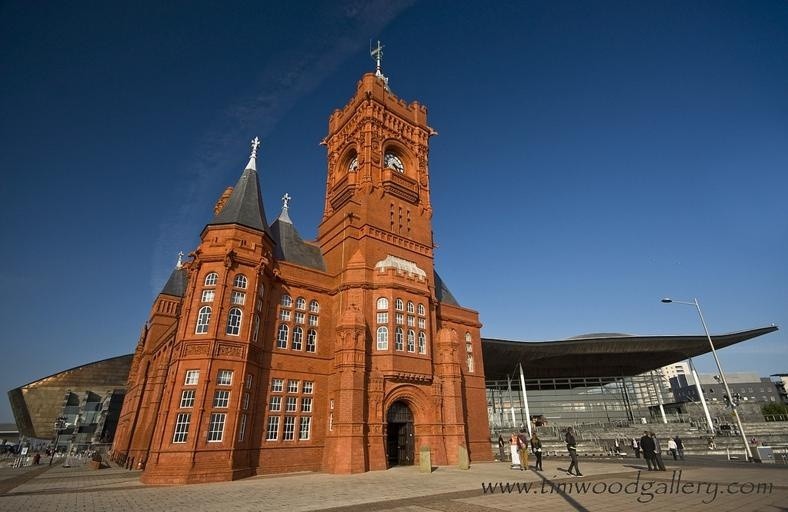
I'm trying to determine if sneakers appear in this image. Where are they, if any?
[648,467,666,471]
[567,471,583,477]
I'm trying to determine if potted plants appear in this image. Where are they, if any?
[90,455,102,468]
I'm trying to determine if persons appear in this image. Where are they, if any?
[529,433,543,471]
[498,434,507,462]
[565,427,584,477]
[610,416,740,473]
[516,429,528,471]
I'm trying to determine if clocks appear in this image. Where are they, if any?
[384,152,404,174]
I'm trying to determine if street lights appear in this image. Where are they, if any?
[660,297,756,464]
